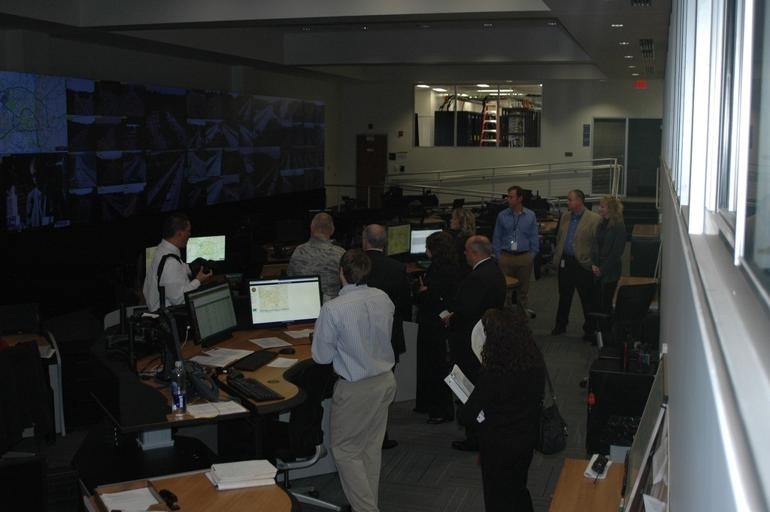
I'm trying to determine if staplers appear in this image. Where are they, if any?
[158,489,180,510]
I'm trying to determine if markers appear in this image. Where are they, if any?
[619,450,630,512]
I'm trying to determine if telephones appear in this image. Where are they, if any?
[186,370,219,402]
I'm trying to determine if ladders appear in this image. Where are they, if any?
[479,95,500,147]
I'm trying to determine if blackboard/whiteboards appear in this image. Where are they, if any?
[619,353,670,512]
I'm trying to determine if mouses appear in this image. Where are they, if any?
[279,347,295,354]
[227,370,242,380]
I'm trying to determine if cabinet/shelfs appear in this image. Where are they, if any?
[433,105,540,147]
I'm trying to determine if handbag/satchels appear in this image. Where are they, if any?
[535,404,566,453]
[157,304,191,340]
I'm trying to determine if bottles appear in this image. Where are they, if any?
[621,341,630,371]
[169,361,188,416]
[636,344,652,375]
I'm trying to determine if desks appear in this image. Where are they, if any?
[544,455,627,512]
[78,461,306,512]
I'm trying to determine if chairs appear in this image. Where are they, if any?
[267,364,344,512]
[583,281,658,347]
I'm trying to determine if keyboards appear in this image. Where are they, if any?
[228,379,285,401]
[235,350,277,370]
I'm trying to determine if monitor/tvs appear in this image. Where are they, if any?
[184,233,227,264]
[247,274,322,328]
[184,280,239,348]
[162,306,185,373]
[384,223,410,256]
[410,226,443,254]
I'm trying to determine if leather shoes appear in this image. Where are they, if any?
[552,326,566,335]
[428,417,450,424]
[452,440,479,450]
[382,439,399,449]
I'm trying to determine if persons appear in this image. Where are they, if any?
[582,194,627,346]
[548,189,607,337]
[492,186,540,323]
[24,155,63,228]
[285,204,552,512]
[141,212,214,320]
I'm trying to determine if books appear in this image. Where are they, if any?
[182,398,253,419]
